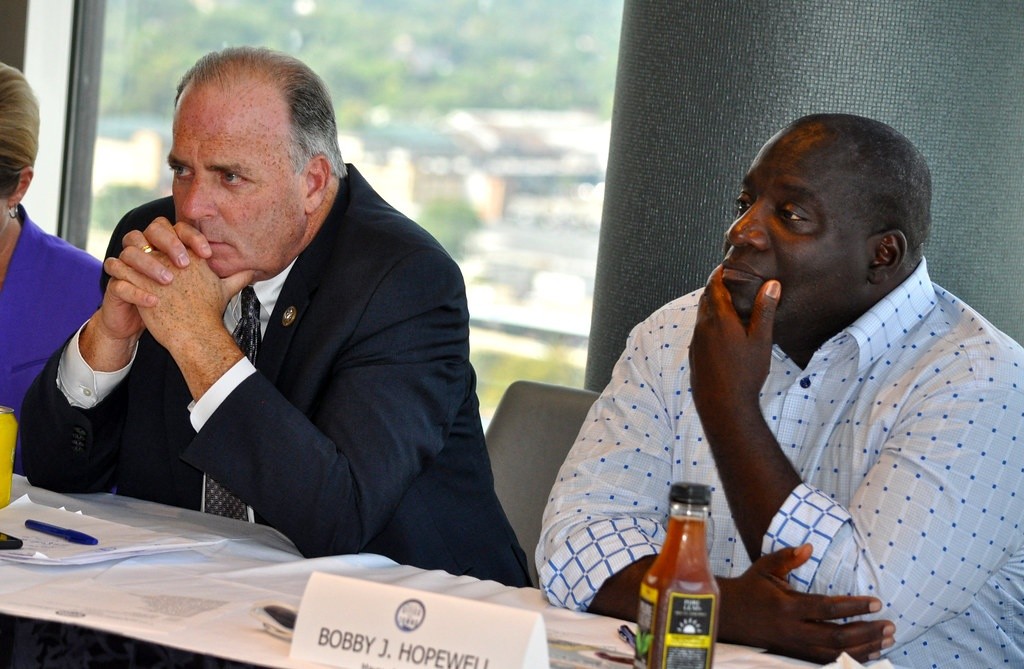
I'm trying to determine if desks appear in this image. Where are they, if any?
[0,465,904,669]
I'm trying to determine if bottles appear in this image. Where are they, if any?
[632,482,721,669]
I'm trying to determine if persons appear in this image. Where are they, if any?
[15,43,535,589]
[0,58,106,478]
[534,112,1024,669]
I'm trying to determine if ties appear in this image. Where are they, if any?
[205,286,261,522]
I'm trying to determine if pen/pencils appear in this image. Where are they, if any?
[25,519,98,546]
[617,623,635,647]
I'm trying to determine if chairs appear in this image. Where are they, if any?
[484,380,602,591]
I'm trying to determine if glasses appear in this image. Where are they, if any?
[250,599,298,640]
[0,165,27,199]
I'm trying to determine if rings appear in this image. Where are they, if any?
[141,246,153,253]
